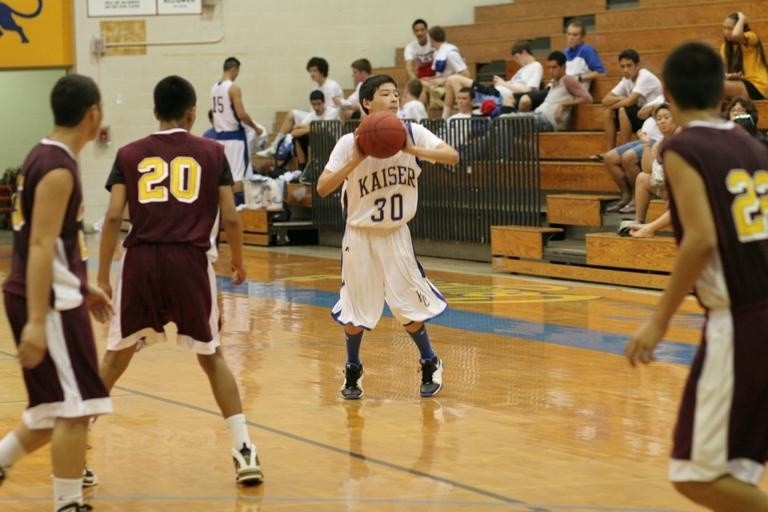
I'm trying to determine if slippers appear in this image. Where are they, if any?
[619,202,636,214]
[606,203,625,214]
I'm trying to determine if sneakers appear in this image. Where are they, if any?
[56,501,92,512]
[81,462,97,487]
[230,442,265,486]
[339,362,367,400]
[416,354,444,398]
[256,143,281,158]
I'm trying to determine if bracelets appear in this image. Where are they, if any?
[578,73,582,82]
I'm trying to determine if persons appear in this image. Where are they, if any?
[503,20,608,112]
[590,49,663,160]
[419,26,470,109]
[604,94,665,214]
[333,58,371,120]
[404,19,437,88]
[317,74,459,399]
[202,109,217,139]
[720,11,768,100]
[725,96,768,146]
[82,75,263,486]
[461,51,593,162]
[290,90,342,171]
[626,42,768,511]
[443,42,543,120]
[256,57,343,157]
[1,75,115,511]
[397,80,429,124]
[619,104,682,237]
[629,208,672,237]
[209,58,263,205]
[446,88,472,140]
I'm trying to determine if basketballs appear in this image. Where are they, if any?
[357,110,405,159]
